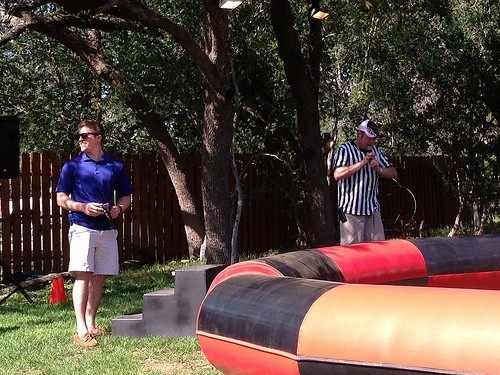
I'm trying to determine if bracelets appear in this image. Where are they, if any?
[379,167,383,175]
[119,205,122,214]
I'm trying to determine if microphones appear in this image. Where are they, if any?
[103,203,112,212]
[367,146,372,153]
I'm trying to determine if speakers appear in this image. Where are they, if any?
[0,115,20,179]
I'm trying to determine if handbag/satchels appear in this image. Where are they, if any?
[50,277,67,304]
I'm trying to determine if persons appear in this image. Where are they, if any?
[56,120,132,348]
[320,119,399,245]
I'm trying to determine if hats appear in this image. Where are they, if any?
[322,133,334,141]
[355,120,379,138]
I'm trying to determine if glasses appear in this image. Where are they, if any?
[76,133,100,141]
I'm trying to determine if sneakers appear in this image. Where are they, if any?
[73,332,98,347]
[90,328,106,337]
